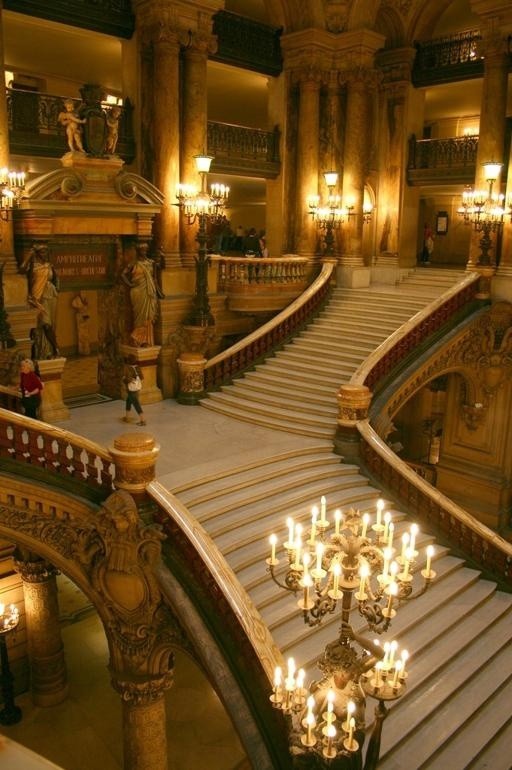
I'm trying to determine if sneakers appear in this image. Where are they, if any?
[136,421,146,426]
[120,417,130,423]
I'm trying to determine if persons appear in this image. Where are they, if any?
[120,355,147,427]
[0,235,18,346]
[421,222,437,264]
[19,242,63,359]
[13,359,43,418]
[104,107,121,154]
[119,241,166,347]
[59,99,86,153]
[235,225,272,285]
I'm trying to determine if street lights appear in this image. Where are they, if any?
[0,603,28,725]
[1,164,26,348]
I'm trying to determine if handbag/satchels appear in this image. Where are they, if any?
[22,396,41,408]
[128,376,142,392]
